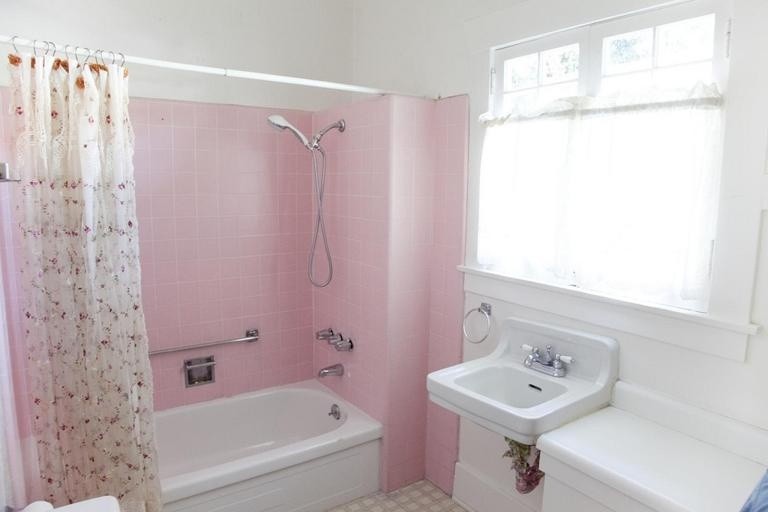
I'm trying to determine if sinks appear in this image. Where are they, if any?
[425,316,619,444]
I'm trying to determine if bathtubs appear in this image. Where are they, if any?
[154,389,348,512]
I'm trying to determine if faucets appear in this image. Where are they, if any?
[519,344,573,377]
[318,364,344,378]
